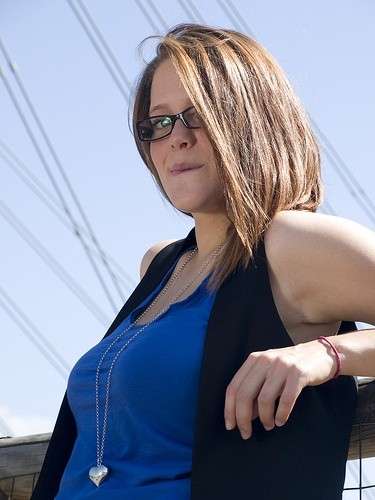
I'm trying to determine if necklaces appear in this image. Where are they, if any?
[88,233,230,488]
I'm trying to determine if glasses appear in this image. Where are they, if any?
[135,105,205,142]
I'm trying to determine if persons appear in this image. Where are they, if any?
[30,24,375,500]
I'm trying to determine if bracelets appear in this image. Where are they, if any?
[317,334,340,379]
